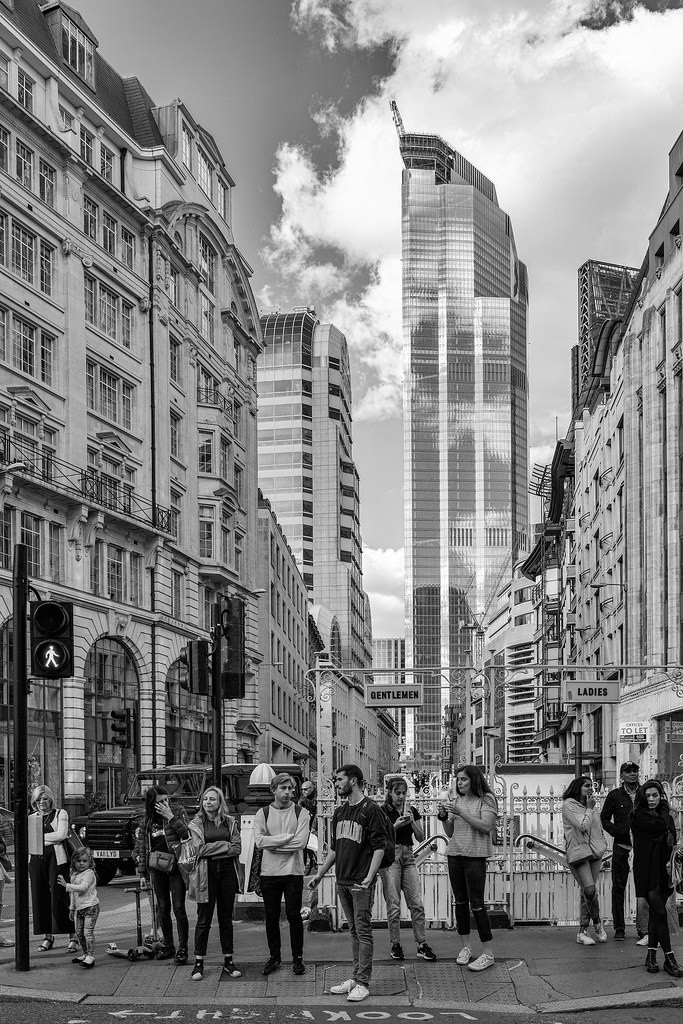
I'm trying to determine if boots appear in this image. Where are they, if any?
[645,948,683,977]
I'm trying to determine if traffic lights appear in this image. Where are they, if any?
[177,639,208,696]
[30,599,75,681]
[111,708,131,748]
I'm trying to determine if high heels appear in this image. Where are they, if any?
[37,934,55,952]
[66,938,80,953]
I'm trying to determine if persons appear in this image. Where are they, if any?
[379,778,436,962]
[252,772,312,975]
[56,846,101,968]
[626,782,683,977]
[29,784,80,954]
[636,779,680,946]
[561,776,607,946]
[298,780,317,869]
[137,785,189,966]
[0,807,16,947]
[306,764,387,1002]
[436,765,499,972]
[600,761,647,942]
[186,786,242,980]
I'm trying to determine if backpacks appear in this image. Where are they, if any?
[366,800,396,870]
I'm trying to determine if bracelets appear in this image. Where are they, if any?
[437,811,449,821]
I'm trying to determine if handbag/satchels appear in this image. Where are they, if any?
[0,844,13,872]
[149,851,175,873]
[177,837,197,891]
[567,843,594,866]
[63,829,84,864]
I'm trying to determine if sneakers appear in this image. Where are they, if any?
[416,943,436,960]
[456,947,495,971]
[390,942,405,959]
[330,979,369,1002]
[262,956,306,974]
[576,917,607,945]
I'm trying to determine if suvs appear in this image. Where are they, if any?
[74,763,304,887]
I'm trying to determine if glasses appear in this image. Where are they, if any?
[301,787,311,790]
[626,770,636,773]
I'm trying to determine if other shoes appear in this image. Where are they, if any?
[223,956,242,978]
[636,934,649,946]
[0,934,15,948]
[613,930,626,941]
[153,944,189,964]
[191,961,204,981]
[72,954,95,968]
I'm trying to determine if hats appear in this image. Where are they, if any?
[621,760,639,772]
